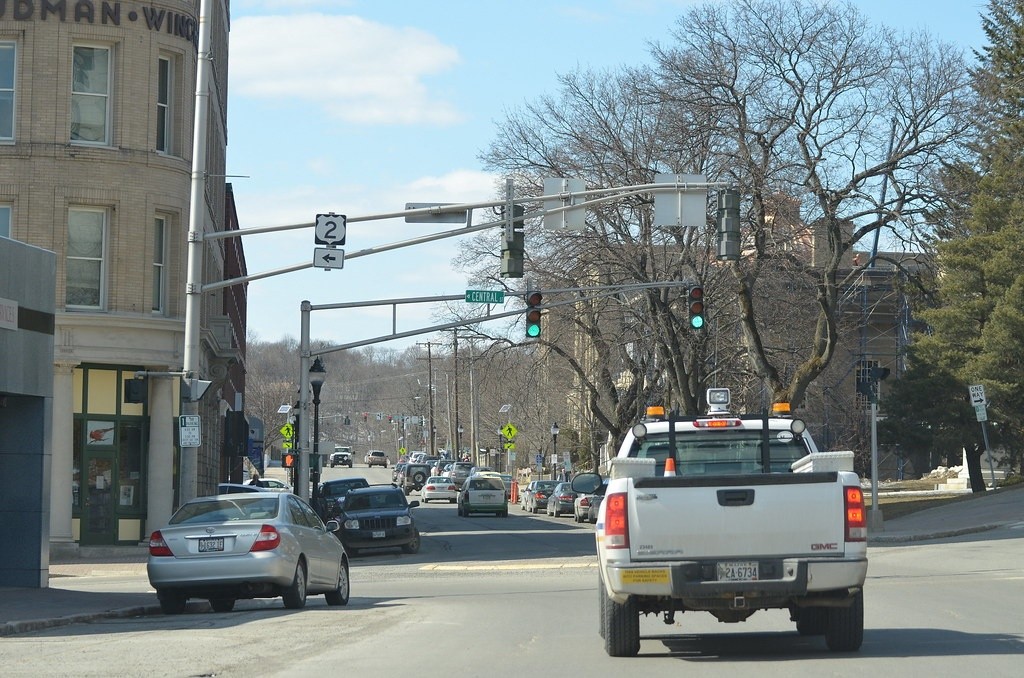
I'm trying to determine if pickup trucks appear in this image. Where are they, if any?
[570,388,874,658]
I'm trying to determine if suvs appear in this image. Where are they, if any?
[330,445,356,468]
[318,478,370,518]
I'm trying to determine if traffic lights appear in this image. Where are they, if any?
[869,366,891,381]
[362,412,368,423]
[387,416,393,424]
[524,291,541,338]
[182,376,214,405]
[688,285,706,330]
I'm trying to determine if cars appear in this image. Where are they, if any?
[146,491,350,616]
[458,475,509,517]
[218,477,313,498]
[520,480,609,525]
[364,449,390,467]
[420,475,457,504]
[391,450,521,500]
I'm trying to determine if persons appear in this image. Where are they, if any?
[249,474,263,487]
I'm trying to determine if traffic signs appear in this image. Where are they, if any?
[968,384,986,408]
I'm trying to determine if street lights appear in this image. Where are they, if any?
[327,483,421,555]
[307,355,329,512]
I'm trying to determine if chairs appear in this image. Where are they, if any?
[385,495,400,508]
[757,441,793,472]
[646,444,682,478]
